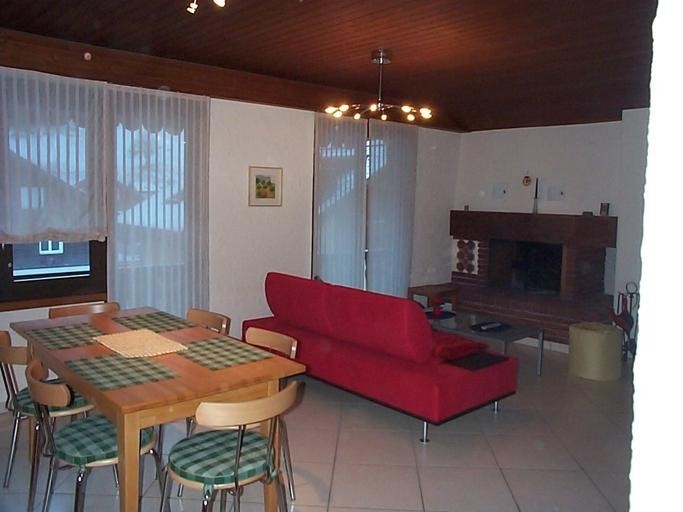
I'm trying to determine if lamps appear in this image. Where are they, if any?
[324,48,432,122]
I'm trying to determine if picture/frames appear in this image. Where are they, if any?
[248,166,282,207]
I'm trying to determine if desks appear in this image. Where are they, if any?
[9,306,307,511]
[422,304,545,376]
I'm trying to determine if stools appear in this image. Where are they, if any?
[567,322,623,382]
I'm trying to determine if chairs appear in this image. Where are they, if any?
[0,302,298,511]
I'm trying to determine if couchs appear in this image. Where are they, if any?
[241,272,518,442]
